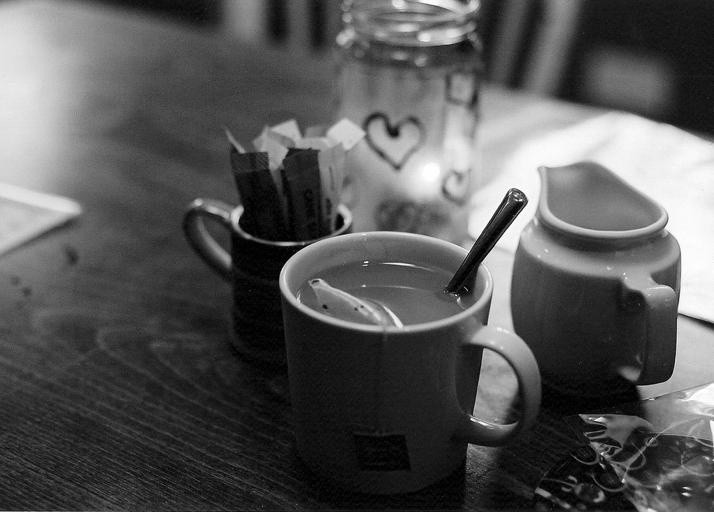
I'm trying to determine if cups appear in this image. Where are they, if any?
[277,229,544,500]
[177,192,356,357]
[507,159,684,389]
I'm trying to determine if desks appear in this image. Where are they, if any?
[0,0,714,511]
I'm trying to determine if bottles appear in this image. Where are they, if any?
[325,0,490,249]
